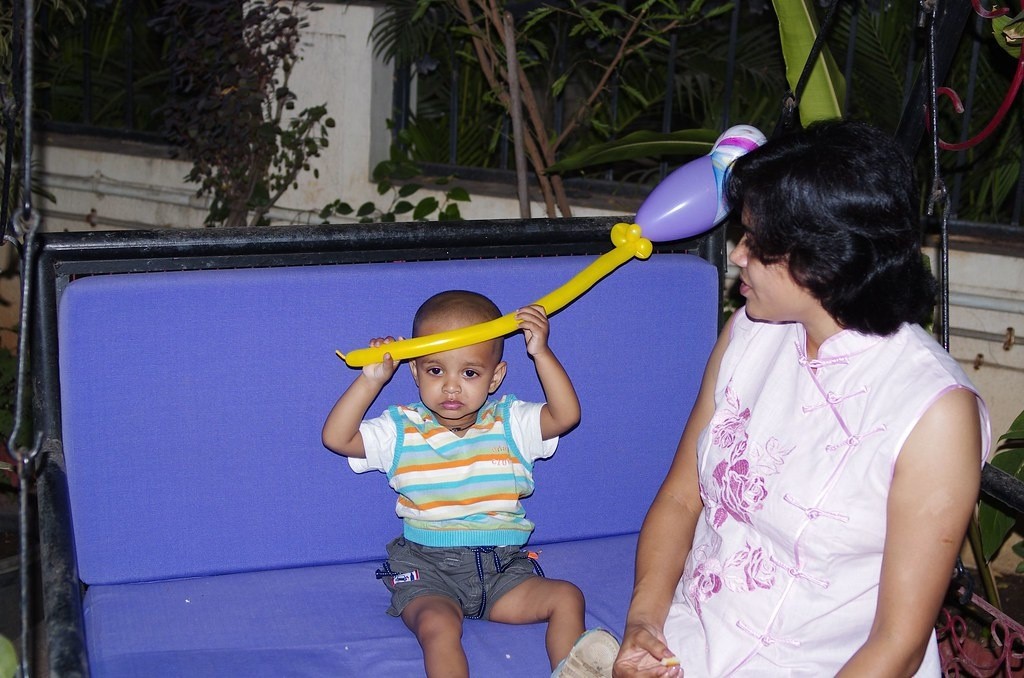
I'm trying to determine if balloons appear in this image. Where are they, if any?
[340,124,765,366]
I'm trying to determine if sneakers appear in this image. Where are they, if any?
[550,627,621,678]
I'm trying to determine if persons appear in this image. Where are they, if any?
[611,117,990,678]
[322,289,621,678]
